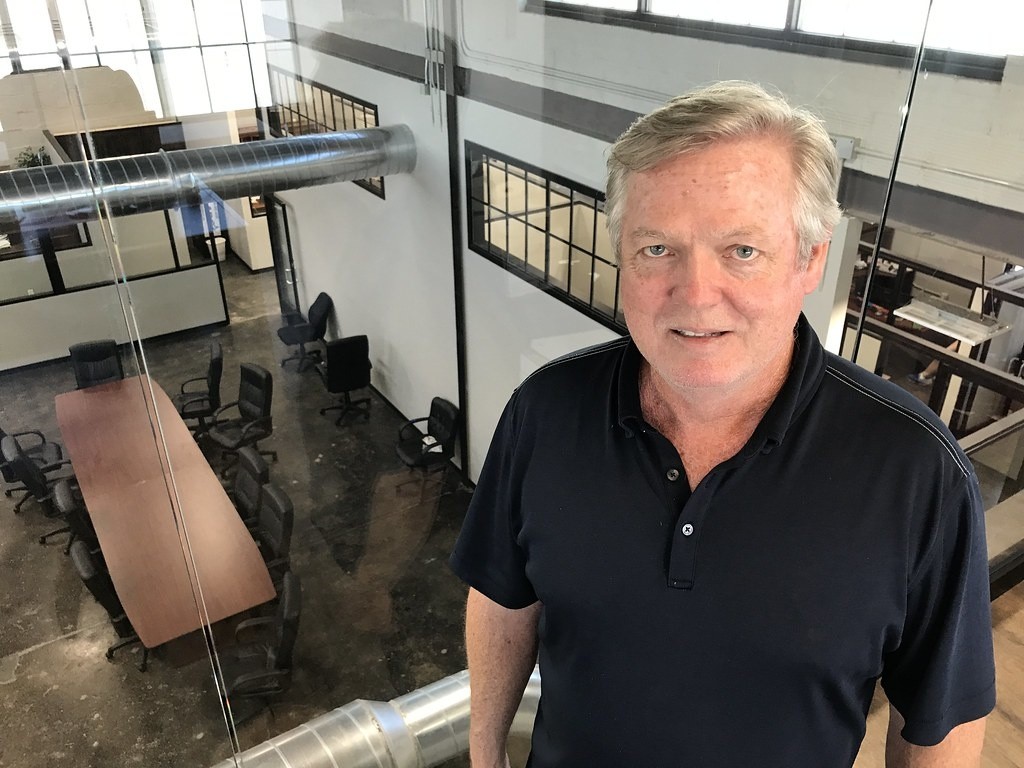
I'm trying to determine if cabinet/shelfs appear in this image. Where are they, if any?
[852,264,916,311]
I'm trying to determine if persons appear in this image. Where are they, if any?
[447,80,995,768]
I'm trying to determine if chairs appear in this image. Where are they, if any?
[277,292,331,372]
[395,397,460,504]
[320,335,373,427]
[0,339,302,730]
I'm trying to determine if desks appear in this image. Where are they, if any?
[54,373,277,650]
